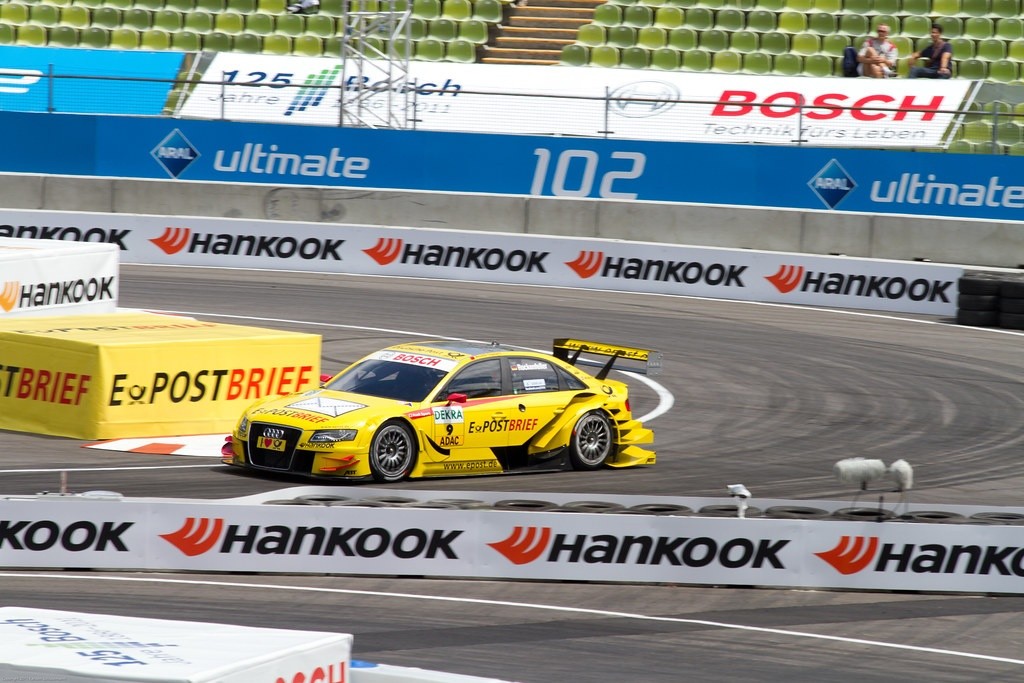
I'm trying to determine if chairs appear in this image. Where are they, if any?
[0,0,1024,160]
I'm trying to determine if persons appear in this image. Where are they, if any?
[509,0,529,8]
[284,0,321,15]
[856,22,899,79]
[906,23,953,79]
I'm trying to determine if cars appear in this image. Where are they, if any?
[230,337,658,483]
